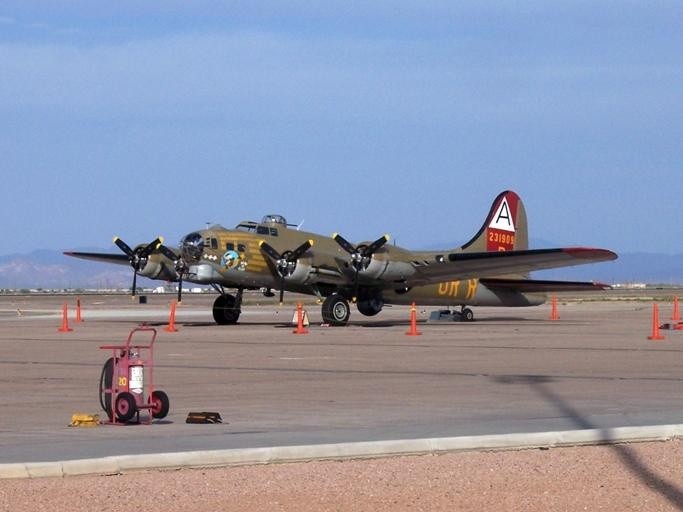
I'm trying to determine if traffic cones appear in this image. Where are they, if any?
[163,301,176,331]
[404,302,422,335]
[59,302,72,331]
[290,302,309,334]
[550,294,560,321]
[668,296,680,320]
[72,296,85,323]
[647,303,664,340]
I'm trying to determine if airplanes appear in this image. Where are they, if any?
[62,190,618,326]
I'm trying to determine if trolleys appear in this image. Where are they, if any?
[96,328,170,425]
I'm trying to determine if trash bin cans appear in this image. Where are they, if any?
[139,296,146,304]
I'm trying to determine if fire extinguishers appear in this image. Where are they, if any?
[128,352,144,407]
